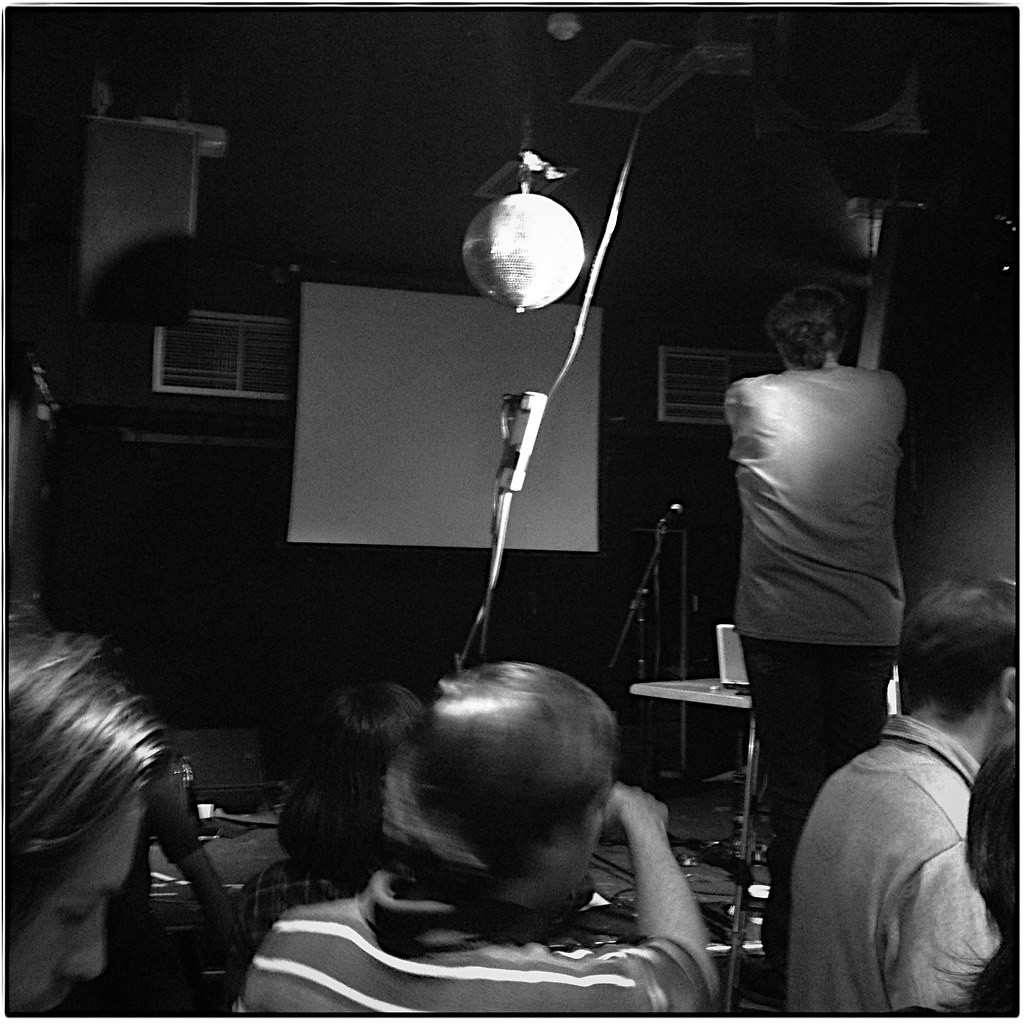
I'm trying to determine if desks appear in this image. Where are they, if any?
[630,677,765,799]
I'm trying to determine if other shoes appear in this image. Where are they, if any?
[740,975,787,1008]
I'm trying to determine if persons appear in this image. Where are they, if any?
[6,579,1018,1013]
[724,280,908,1009]
